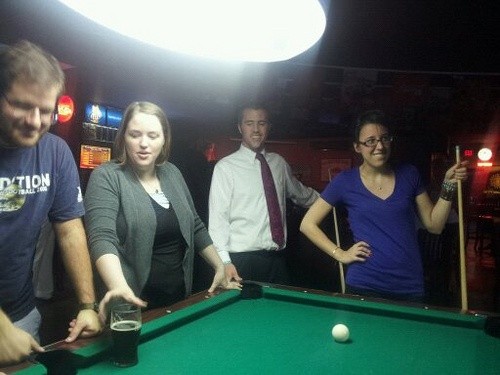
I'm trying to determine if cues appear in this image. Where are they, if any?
[327,168,346,294]
[455,144,468,311]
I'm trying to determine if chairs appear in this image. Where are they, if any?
[464,197,497,256]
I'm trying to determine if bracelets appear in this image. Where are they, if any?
[439,180,457,201]
[332,247,340,258]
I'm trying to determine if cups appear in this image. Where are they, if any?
[109,303,142,368]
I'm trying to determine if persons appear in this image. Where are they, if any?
[208,103,320,283]
[83,101,242,307]
[0,36,104,366]
[300,110,468,304]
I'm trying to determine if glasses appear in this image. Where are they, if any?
[359,135,393,147]
[1,91,58,124]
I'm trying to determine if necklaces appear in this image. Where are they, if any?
[141,169,159,194]
[362,172,382,191]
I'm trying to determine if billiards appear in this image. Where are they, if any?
[332,324,350,342]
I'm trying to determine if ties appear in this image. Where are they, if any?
[255,152,285,247]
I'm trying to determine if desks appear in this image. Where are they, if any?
[0,281,500,375]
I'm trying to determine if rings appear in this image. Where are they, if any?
[461,173,465,177]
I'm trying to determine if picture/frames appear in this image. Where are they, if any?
[320,158,354,182]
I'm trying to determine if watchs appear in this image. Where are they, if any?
[78,301,100,314]
